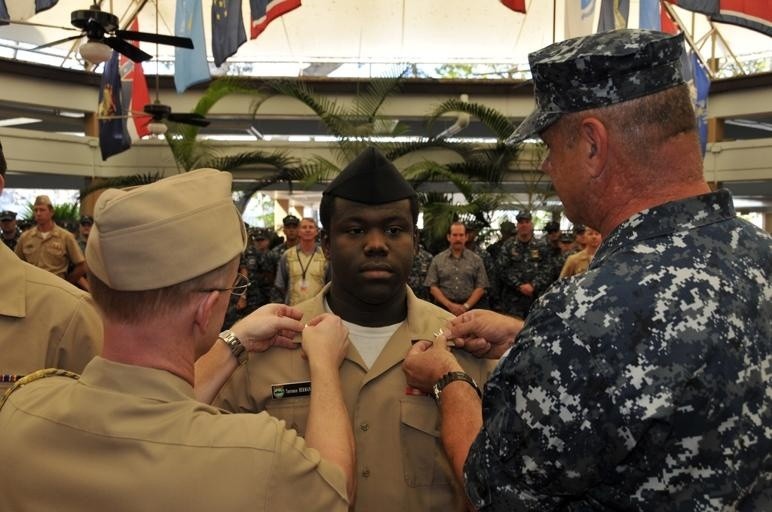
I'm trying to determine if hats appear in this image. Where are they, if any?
[17,215,93,232]
[499,210,586,244]
[501,27,687,151]
[0,209,17,222]
[252,229,272,242]
[320,143,421,208]
[33,193,53,208]
[83,165,251,297]
[282,215,300,227]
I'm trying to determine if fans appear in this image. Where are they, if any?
[94,0,210,128]
[0,0,194,63]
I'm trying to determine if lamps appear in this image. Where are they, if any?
[77,40,114,64]
[147,121,167,135]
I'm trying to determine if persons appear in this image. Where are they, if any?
[403,31,772,510]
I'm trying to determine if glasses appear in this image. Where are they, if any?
[188,270,251,298]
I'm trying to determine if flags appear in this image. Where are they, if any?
[211,1,248,67]
[119,18,153,143]
[98,43,130,162]
[173,0,210,93]
[249,0,302,39]
